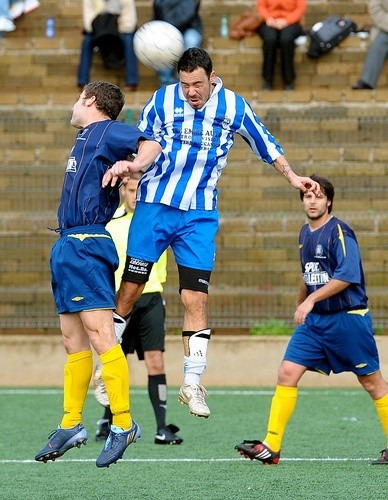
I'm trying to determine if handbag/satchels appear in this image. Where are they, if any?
[307,17,356,59]
[228,12,260,40]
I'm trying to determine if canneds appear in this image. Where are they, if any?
[125,110,135,123]
[220,16,229,38]
[45,17,55,39]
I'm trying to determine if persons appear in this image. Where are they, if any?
[152,0,203,88]
[256,0,308,90]
[77,0,138,94]
[92,46,320,417]
[352,0,387,90]
[0,0,42,34]
[33,79,164,469]
[96,171,186,445]
[234,171,387,466]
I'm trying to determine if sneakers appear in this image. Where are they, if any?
[373,448,388,464]
[352,81,372,88]
[92,365,110,406]
[178,383,211,417]
[284,82,294,89]
[263,79,272,89]
[96,419,141,467]
[234,440,281,464]
[95,419,111,441]
[155,424,183,445]
[35,423,87,463]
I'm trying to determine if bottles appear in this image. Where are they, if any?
[220,14,229,39]
[45,16,55,38]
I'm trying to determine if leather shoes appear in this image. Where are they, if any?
[122,86,136,92]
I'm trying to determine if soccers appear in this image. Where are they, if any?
[133,20,184,71]
